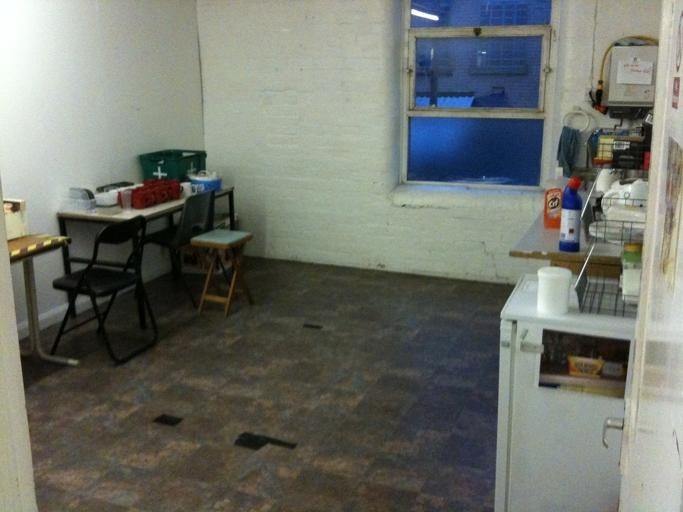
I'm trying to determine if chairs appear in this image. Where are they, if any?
[136,187,218,310]
[49,214,159,365]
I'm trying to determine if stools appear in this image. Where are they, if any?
[190,229,254,317]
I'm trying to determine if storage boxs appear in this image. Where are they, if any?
[138,149,207,183]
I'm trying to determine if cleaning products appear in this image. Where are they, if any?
[544,168,562,228]
[559,176,582,252]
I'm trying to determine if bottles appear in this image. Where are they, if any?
[544,167,569,229]
[622,242,641,265]
[560,177,582,252]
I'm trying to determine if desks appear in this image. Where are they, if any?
[57,186,240,332]
